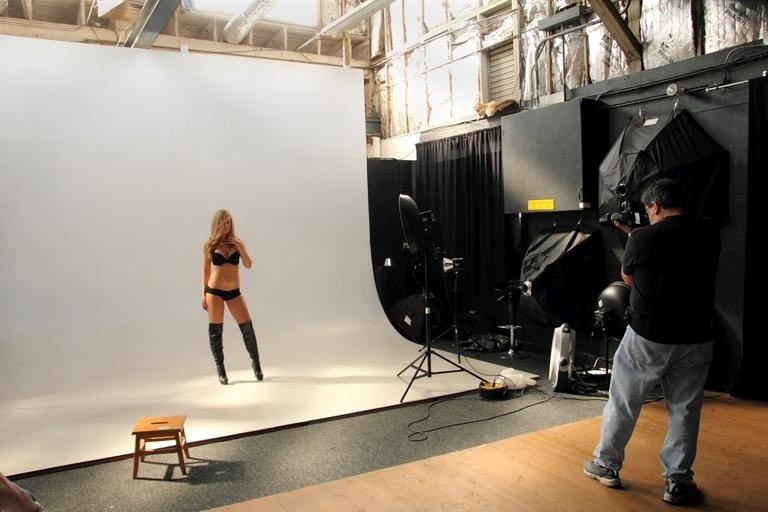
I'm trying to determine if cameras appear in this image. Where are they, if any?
[598,199,650,231]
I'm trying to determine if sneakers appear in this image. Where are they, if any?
[583,461,619,486]
[663,482,698,502]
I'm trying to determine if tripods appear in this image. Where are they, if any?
[396,227,489,403]
[418,271,487,364]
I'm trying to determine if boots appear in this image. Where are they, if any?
[209,323,228,384]
[240,322,263,380]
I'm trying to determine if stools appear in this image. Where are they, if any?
[131,416,190,479]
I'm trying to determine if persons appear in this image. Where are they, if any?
[583,179,717,505]
[200,209,263,386]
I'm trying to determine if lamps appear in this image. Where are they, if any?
[397,193,488,403]
